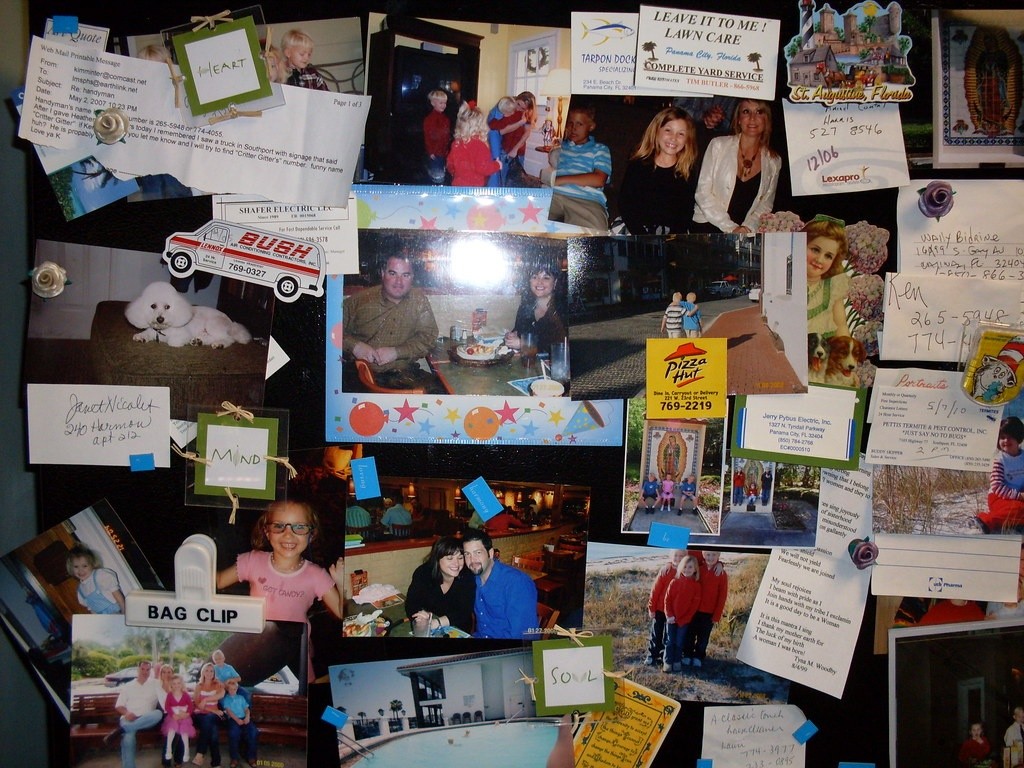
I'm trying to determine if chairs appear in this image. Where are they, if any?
[512,555,545,574]
[450,709,483,725]
[353,355,426,393]
[536,604,561,639]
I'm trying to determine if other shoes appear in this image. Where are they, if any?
[693,658,702,667]
[230,760,241,768]
[663,663,672,672]
[192,756,204,765]
[651,507,654,513]
[691,509,698,515]
[682,658,690,665]
[646,506,649,514]
[644,656,652,664]
[250,759,257,768]
[674,662,681,671]
[104,729,119,747]
[678,510,681,515]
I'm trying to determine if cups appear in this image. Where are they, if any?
[520,333,538,369]
[529,378,565,397]
[551,342,571,381]
[412,617,431,637]
[547,545,554,552]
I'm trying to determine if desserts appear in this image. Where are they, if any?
[456,342,496,361]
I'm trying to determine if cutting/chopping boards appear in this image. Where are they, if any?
[448,344,514,366]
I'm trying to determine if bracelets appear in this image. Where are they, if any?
[436,618,441,630]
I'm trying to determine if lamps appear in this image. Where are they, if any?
[407,481,416,499]
[535,69,572,154]
[516,490,522,502]
[453,483,462,501]
[349,479,355,495]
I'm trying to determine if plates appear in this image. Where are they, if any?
[344,613,393,637]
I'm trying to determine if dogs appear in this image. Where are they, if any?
[124,281,251,349]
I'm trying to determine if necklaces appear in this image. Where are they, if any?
[739,139,760,177]
[271,552,303,572]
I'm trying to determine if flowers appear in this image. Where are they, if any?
[918,180,958,225]
[22,259,72,300]
[88,109,132,147]
[847,534,880,572]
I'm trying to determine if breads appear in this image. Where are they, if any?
[344,624,370,636]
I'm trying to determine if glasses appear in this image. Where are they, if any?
[265,523,313,535]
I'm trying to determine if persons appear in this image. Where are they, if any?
[216,499,345,683]
[644,549,728,672]
[550,99,851,337]
[423,90,537,187]
[345,487,539,640]
[281,29,328,92]
[639,467,773,515]
[1003,706,1024,763]
[259,38,286,84]
[65,547,125,615]
[975,416,1024,533]
[893,543,1024,630]
[341,252,445,394]
[501,257,569,358]
[959,721,990,768]
[103,648,259,768]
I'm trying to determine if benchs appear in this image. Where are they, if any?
[70,694,307,754]
[638,486,698,510]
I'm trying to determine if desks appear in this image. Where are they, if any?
[343,592,438,637]
[429,335,554,401]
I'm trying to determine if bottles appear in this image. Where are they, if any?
[450,325,468,340]
